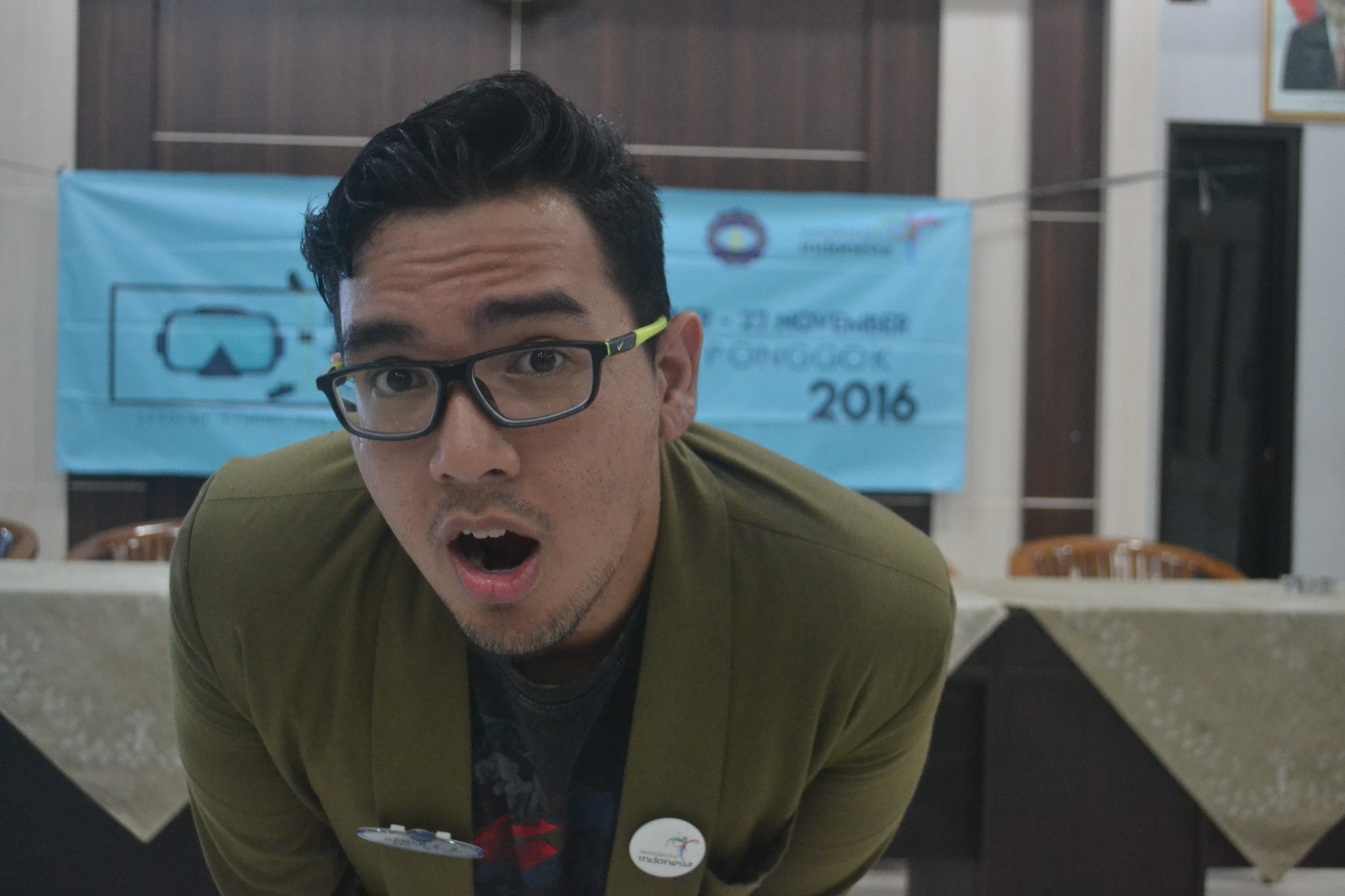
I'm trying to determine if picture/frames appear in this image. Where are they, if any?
[1255,1,1345,126]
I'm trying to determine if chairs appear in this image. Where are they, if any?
[1011,534,1250,592]
[0,513,194,569]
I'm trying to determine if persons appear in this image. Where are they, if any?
[156,65,959,890]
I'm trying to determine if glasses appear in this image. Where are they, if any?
[314,317,669,442]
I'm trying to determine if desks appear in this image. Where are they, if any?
[854,579,1345,896]
[0,558,223,896]
